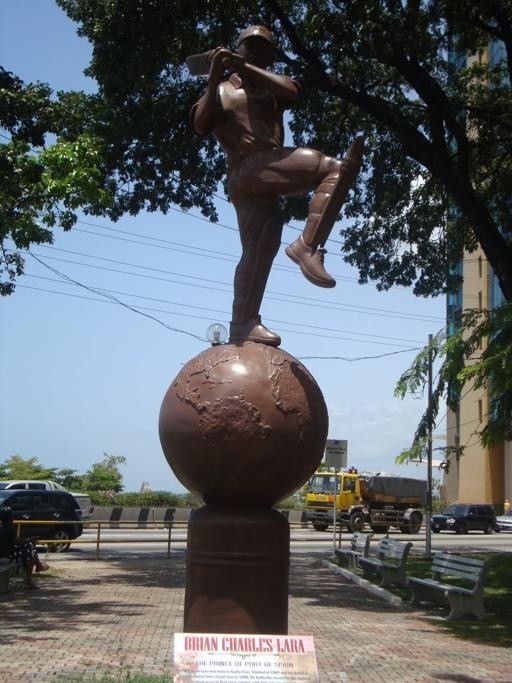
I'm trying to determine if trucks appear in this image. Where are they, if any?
[300,466,429,535]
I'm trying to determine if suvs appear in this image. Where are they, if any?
[0,489,84,553]
[431,500,496,536]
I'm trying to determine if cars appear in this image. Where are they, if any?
[0,477,95,522]
[495,508,512,533]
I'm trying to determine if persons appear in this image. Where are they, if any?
[188,22,366,346]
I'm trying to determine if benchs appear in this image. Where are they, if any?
[333,532,489,624]
[0,559,35,592]
